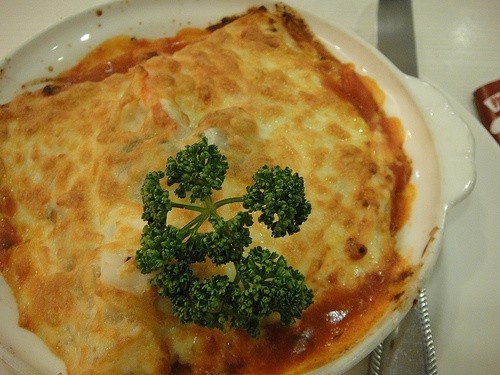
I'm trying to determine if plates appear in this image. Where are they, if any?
[0,0,475,375]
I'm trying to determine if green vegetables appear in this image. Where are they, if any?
[133,138,316,336]
